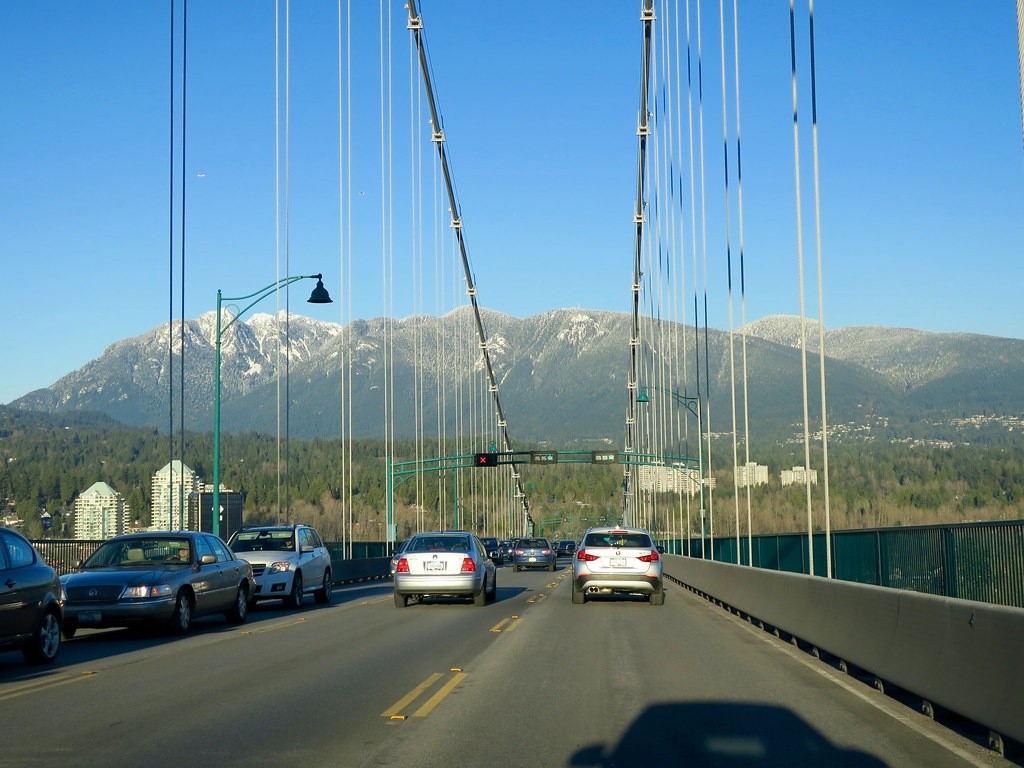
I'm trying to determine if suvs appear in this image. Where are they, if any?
[573,527,664,606]
[552,540,576,557]
[217,523,332,608]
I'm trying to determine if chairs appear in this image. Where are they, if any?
[121,549,149,564]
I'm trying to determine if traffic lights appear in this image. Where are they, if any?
[563,518,569,522]
[474,453,497,467]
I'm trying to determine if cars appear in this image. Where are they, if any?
[513,538,557,572]
[59,532,257,639]
[480,538,504,566]
[499,540,514,562]
[393,532,496,608]
[0,527,63,664]
[391,539,411,575]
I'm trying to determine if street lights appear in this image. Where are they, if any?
[454,441,498,530]
[636,386,706,536]
[213,272,333,536]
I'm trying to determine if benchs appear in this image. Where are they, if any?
[414,546,466,550]
[594,541,644,547]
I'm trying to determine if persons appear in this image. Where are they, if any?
[170,549,189,561]
[281,537,292,548]
